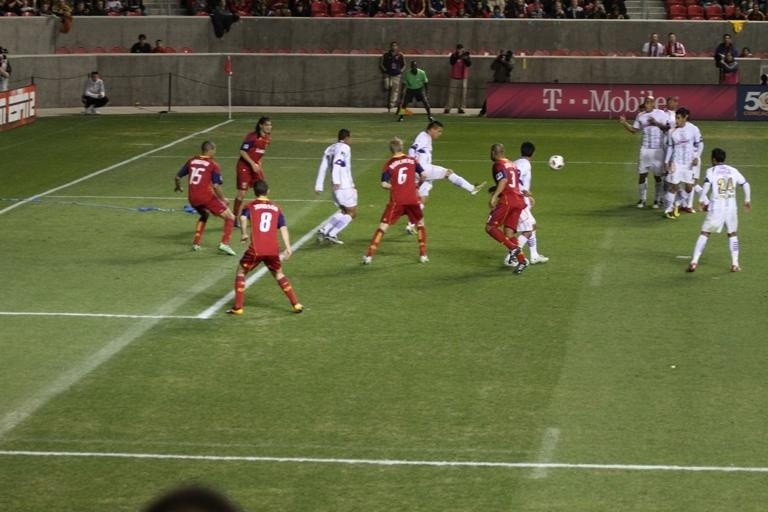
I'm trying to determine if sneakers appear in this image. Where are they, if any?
[217,241,237,256]
[226,309,243,313]
[316,229,344,246]
[470,181,487,195]
[399,109,466,122]
[406,223,417,235]
[419,256,428,263]
[687,263,697,270]
[293,302,304,313]
[505,253,549,273]
[731,265,742,272]
[362,255,372,265]
[636,199,709,218]
[192,245,200,251]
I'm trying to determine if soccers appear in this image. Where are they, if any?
[549,155,565,170]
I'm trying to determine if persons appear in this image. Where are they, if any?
[311,131,359,248]
[227,181,304,315]
[173,142,236,256]
[81,71,109,113]
[478,48,514,117]
[231,116,272,230]
[380,40,403,113]
[663,32,686,59]
[405,122,487,236]
[698,0,767,21]
[687,147,752,272]
[505,141,549,265]
[443,44,472,114]
[0,46,12,92]
[642,32,663,58]
[714,51,740,85]
[617,92,703,219]
[740,47,751,57]
[2,0,631,20]
[398,60,434,123]
[363,138,431,265]
[130,34,151,52]
[153,39,167,53]
[487,142,529,276]
[716,34,737,58]
